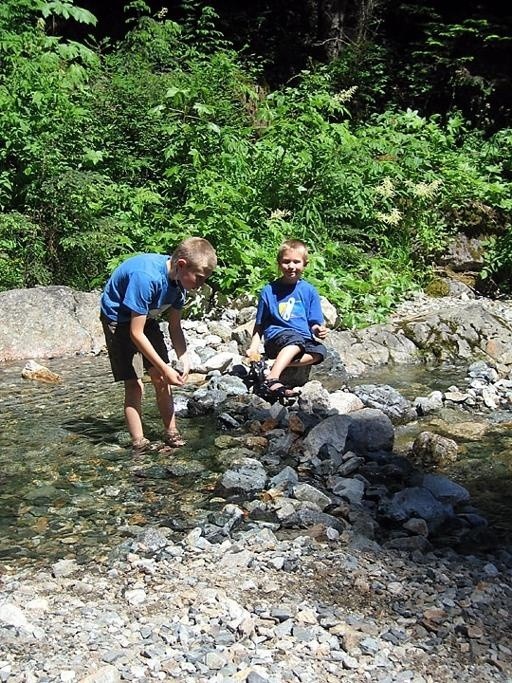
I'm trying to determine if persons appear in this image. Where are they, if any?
[98,235,218,455]
[246,238,331,398]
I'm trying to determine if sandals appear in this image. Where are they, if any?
[132,432,185,453]
[250,361,302,397]
